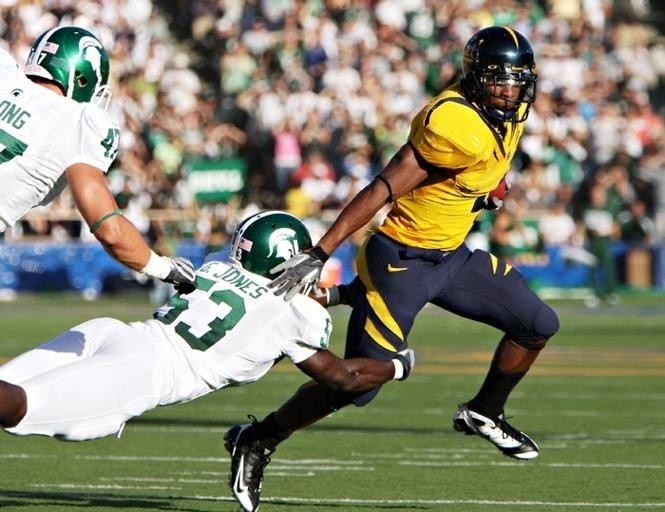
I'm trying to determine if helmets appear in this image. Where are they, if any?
[24,27,112,112]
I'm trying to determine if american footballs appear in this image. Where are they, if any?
[489,176,506,201]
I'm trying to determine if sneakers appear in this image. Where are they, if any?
[454,402,540,459]
[223,421,275,508]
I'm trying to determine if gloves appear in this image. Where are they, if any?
[392,348,414,381]
[337,275,362,307]
[162,255,197,294]
[268,245,329,301]
